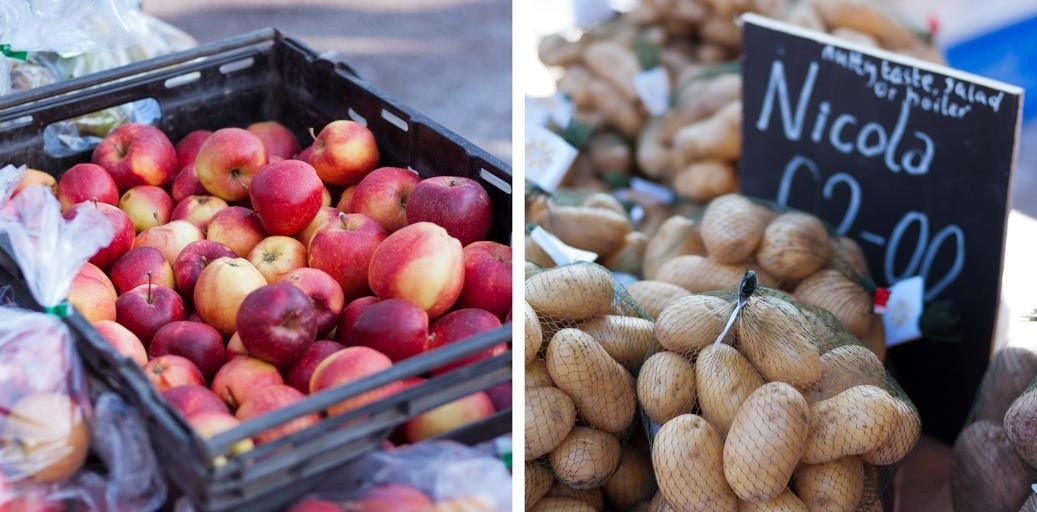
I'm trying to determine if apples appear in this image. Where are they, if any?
[283,484,441,511]
[0,118,511,469]
[0,323,166,512]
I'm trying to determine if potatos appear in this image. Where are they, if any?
[526,3,952,512]
[951,348,1037,511]
